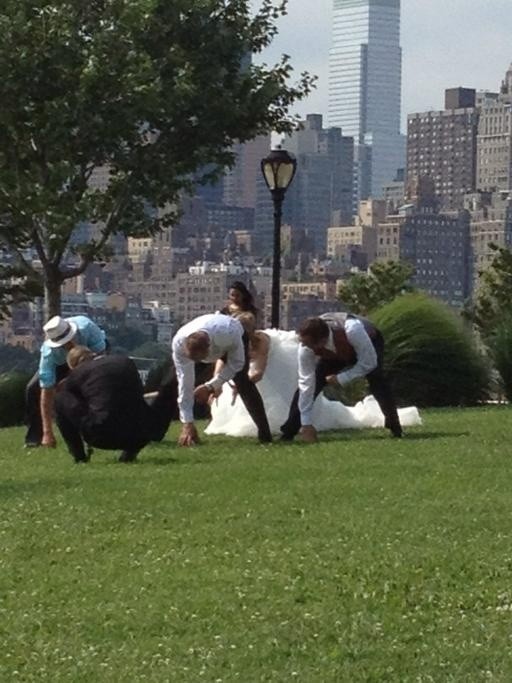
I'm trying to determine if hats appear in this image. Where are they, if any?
[42,315,77,348]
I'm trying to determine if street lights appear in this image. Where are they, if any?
[261,130,296,328]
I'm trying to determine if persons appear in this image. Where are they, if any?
[216,311,270,407]
[149,312,274,451]
[50,343,159,465]
[275,311,402,442]
[215,280,263,329]
[22,314,111,449]
